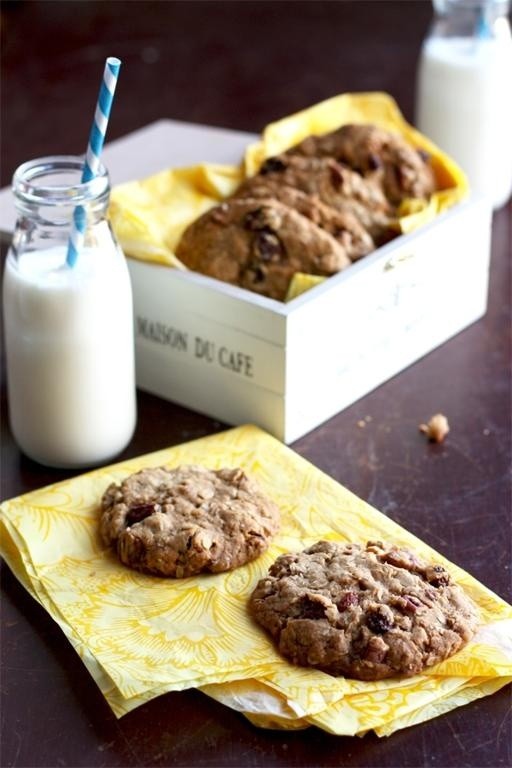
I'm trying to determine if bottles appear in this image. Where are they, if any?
[409,0,512,213]
[1,153,141,471]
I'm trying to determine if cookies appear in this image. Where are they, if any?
[248,538,478,683]
[100,464,281,579]
[175,122,438,302]
